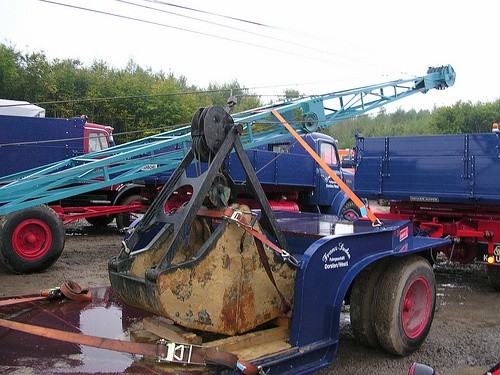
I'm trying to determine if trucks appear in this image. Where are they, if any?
[129,123,363,221]
[1,115,145,226]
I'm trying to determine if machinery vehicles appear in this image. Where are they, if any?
[108,104,451,373]
